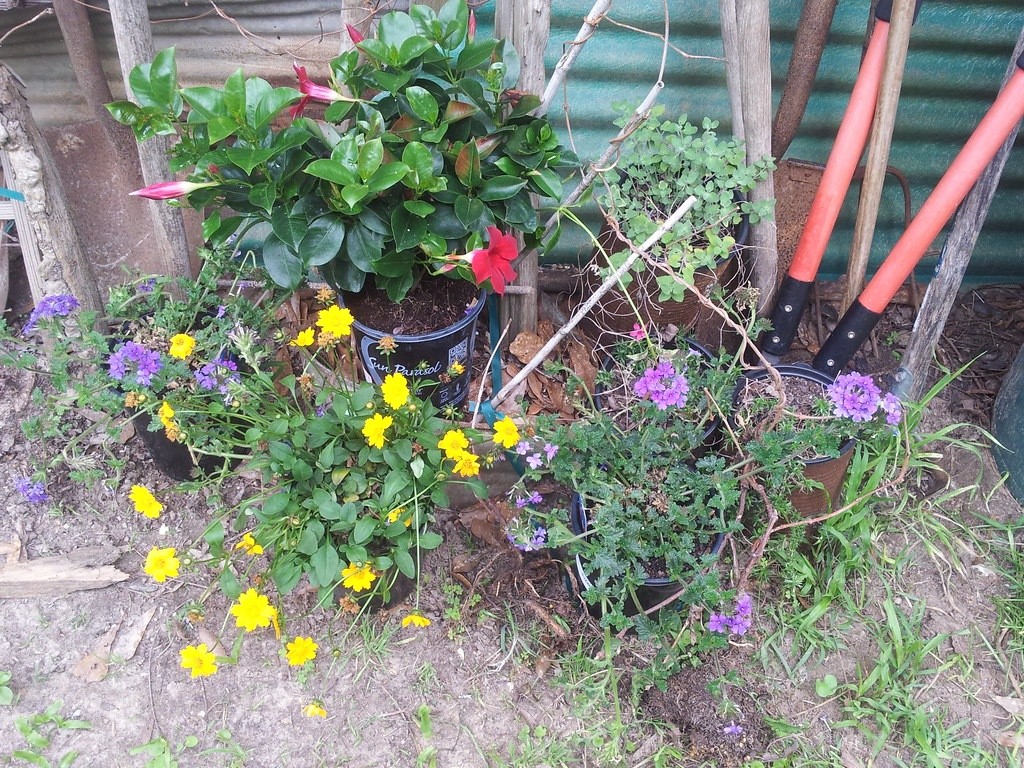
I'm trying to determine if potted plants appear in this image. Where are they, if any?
[578,103,778,349]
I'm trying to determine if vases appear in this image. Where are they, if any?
[334,273,487,416]
[100,312,250,484]
[729,363,859,545]
[571,461,724,635]
[595,334,727,459]
[323,505,428,613]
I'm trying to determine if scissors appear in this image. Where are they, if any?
[756,0,1024,379]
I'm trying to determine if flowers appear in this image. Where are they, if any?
[0,0,903,717]
